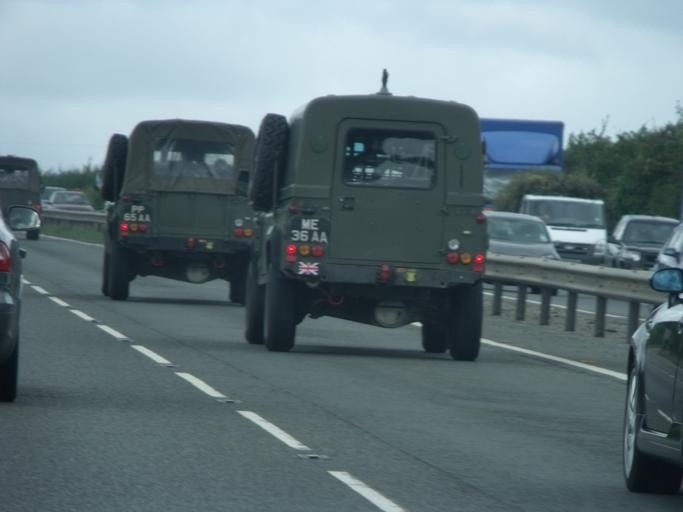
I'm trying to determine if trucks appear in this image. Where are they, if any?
[478,118,566,209]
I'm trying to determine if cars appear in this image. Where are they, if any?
[482,209,562,297]
[40,186,93,212]
[621,269,683,489]
[609,215,681,271]
[0,213,26,401]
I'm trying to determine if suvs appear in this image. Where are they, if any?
[518,194,608,266]
[0,155,44,239]
[247,78,489,363]
[100,121,257,303]
